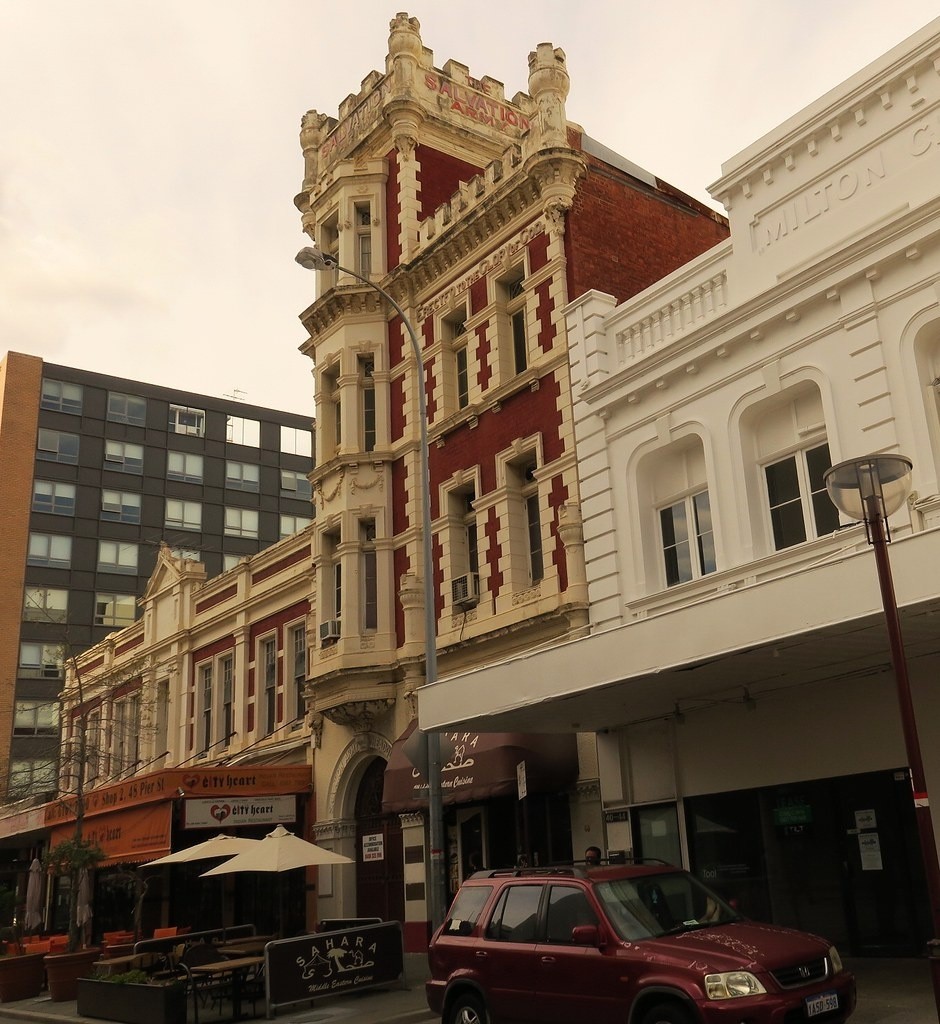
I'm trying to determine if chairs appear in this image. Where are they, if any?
[2,925,191,953]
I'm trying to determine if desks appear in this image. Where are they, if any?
[92,934,274,1021]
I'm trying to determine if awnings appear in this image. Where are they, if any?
[0,796,48,846]
[43,761,315,868]
[372,712,580,815]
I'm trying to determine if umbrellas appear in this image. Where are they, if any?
[23,857,43,943]
[75,861,93,948]
[197,821,357,940]
[134,830,263,946]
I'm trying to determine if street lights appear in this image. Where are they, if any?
[289,247,450,952]
[824,451,940,1021]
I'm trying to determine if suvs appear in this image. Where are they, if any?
[424,856,859,1024]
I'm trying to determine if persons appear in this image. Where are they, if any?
[583,846,602,866]
